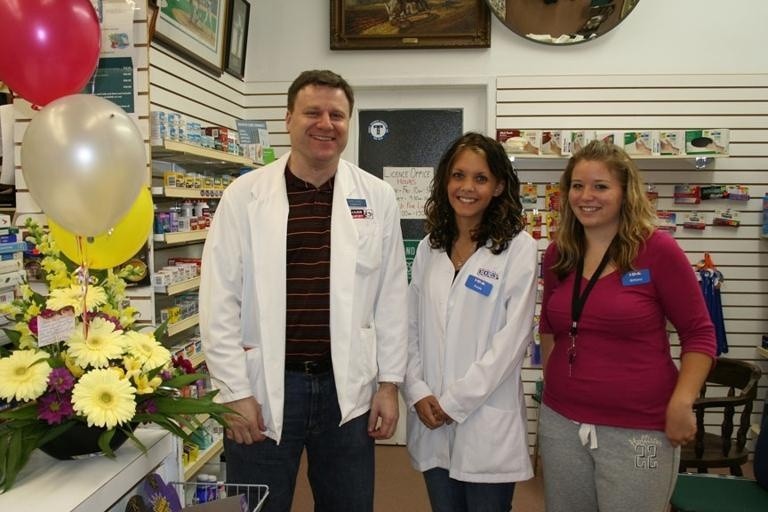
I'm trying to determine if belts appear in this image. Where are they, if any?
[286,360,329,375]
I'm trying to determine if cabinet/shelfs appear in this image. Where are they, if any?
[136,135,268,489]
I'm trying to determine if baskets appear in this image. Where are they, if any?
[167,480,270,511]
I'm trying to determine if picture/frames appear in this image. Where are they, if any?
[148,1,232,77]
[327,1,493,52]
[147,5,159,45]
[223,0,251,82]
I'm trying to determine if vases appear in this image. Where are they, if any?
[25,413,141,463]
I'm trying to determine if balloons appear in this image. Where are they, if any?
[20,93,145,241]
[47,187,155,272]
[1,0,101,107]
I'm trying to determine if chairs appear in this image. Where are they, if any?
[674,352,763,476]
[666,471,768,511]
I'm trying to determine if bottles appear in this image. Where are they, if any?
[182,199,210,229]
[193,472,218,504]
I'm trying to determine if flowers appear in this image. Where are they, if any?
[0,217,245,498]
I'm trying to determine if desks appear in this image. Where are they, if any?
[1,419,187,512]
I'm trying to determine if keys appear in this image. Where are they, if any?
[566,352,575,379]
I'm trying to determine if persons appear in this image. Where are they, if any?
[398,132,539,512]
[197,70,408,512]
[538,139,718,512]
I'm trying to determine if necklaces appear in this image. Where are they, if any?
[454,240,477,268]
